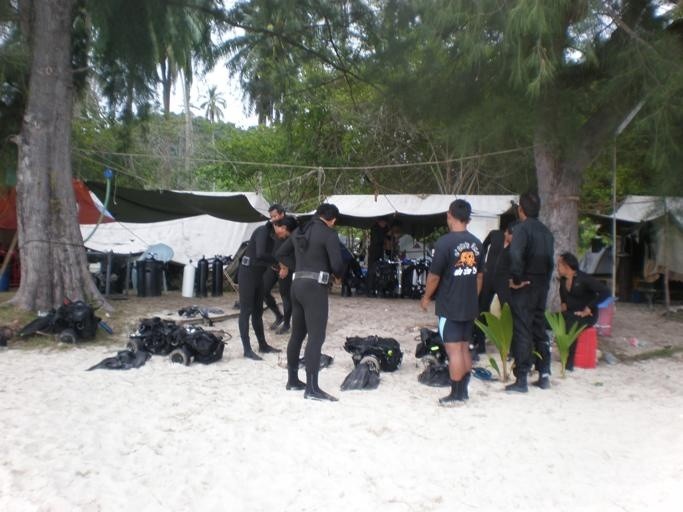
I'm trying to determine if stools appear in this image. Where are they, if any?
[572,327,597,368]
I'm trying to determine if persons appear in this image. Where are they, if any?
[503,190,556,393]
[471,219,516,360]
[366,217,390,298]
[260,205,295,336]
[543,251,610,371]
[237,215,300,361]
[273,202,345,402]
[419,198,486,407]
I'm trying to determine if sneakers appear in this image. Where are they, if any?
[505,375,528,393]
[531,372,550,390]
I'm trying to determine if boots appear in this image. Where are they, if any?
[438,373,471,405]
